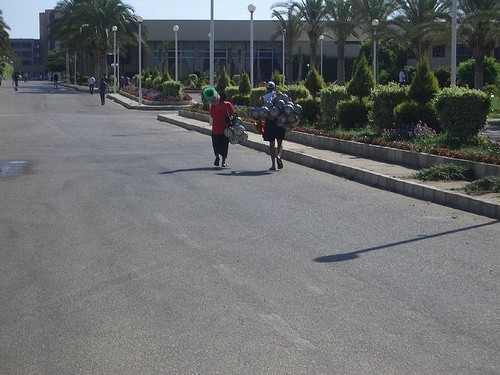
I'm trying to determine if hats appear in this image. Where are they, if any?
[266,81,275,89]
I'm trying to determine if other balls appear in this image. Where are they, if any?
[224,116,249,144]
[247,91,303,132]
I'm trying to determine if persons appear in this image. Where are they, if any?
[88,75,95,94]
[260,81,285,170]
[99,79,107,106]
[210,91,235,166]
[0,72,59,92]
[398,69,410,85]
[104,73,133,90]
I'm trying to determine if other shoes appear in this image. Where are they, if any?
[276,156,283,169]
[222,164,228,167]
[214,158,219,166]
[270,168,275,170]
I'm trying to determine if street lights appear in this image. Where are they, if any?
[112,26,117,92]
[319,34,324,77]
[282,29,287,86]
[173,25,180,81]
[247,4,257,87]
[137,16,144,104]
[372,19,379,80]
